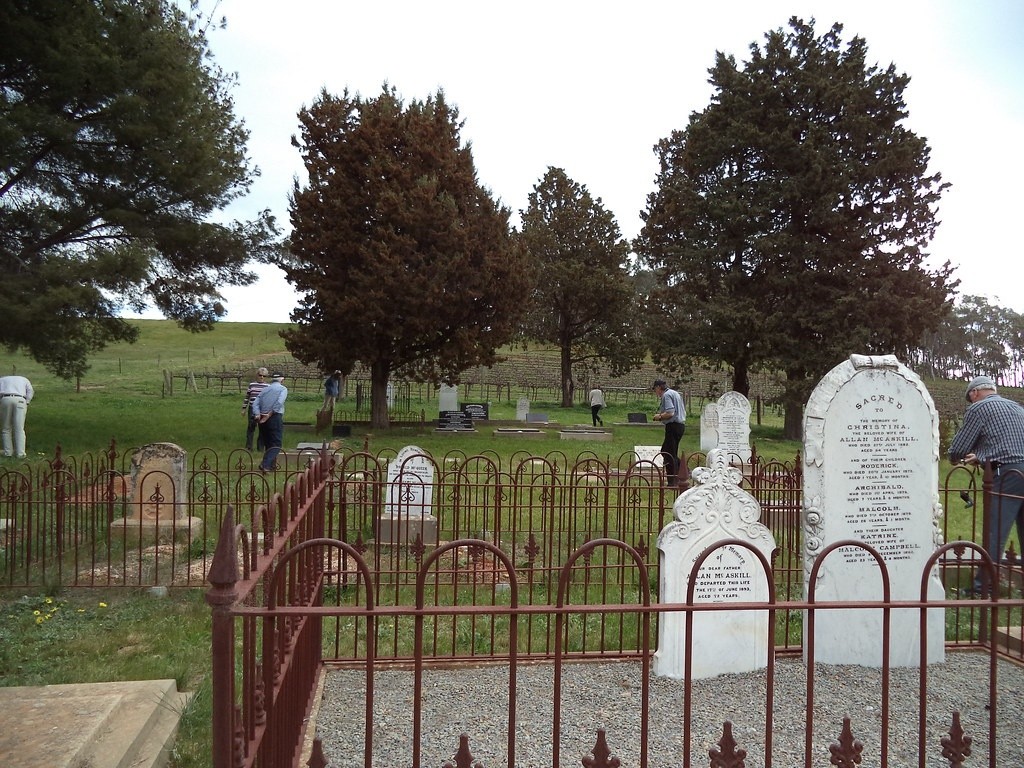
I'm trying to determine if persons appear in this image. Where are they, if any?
[651,379,687,488]
[0,376,34,457]
[320,370,341,412]
[252,371,287,475]
[241,368,271,453]
[948,376,1024,599]
[589,384,607,427]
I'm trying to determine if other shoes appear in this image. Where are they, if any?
[259,465,267,476]
[600,420,603,426]
[17,454,26,457]
[5,455,13,457]
[594,426,596,427]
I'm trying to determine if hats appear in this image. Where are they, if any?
[965,376,996,402]
[652,381,666,390]
[271,372,284,378]
[334,370,343,376]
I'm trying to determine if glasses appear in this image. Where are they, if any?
[258,373,267,377]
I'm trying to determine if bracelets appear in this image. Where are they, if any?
[659,414,662,421]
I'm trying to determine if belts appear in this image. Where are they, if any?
[0,393,27,400]
